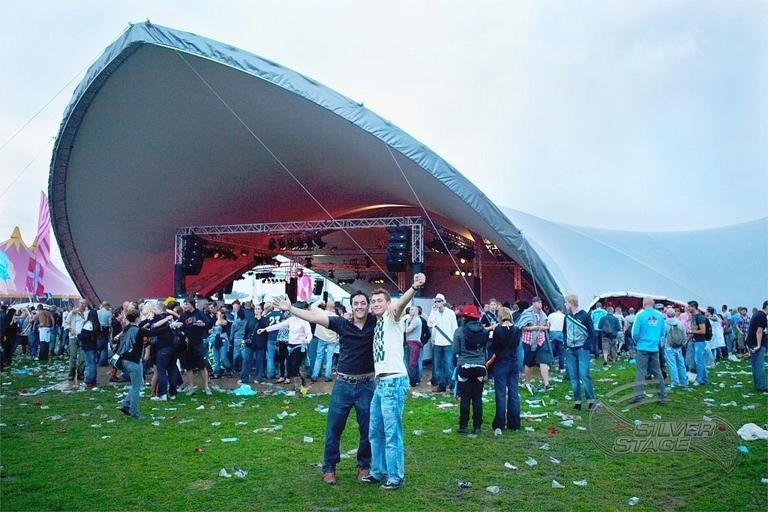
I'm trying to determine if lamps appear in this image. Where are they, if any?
[183,227,476,303]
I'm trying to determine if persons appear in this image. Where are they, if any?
[362,272,427,490]
[1,295,768,432]
[275,292,377,484]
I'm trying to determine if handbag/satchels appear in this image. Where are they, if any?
[669,325,683,347]
[464,320,487,350]
[705,316,712,340]
[109,354,123,370]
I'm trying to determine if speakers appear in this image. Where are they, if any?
[223,281,233,294]
[312,280,323,295]
[386,226,410,272]
[285,279,297,303]
[181,236,207,276]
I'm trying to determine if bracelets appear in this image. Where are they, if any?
[411,284,419,291]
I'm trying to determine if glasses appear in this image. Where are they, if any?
[434,300,440,303]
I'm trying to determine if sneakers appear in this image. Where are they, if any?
[323,471,337,484]
[358,469,404,489]
[150,395,168,401]
[185,387,195,396]
[117,404,131,417]
[204,386,212,396]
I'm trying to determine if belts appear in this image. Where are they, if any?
[337,373,374,385]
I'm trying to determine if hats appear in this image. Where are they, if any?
[457,305,481,318]
[433,294,446,303]
